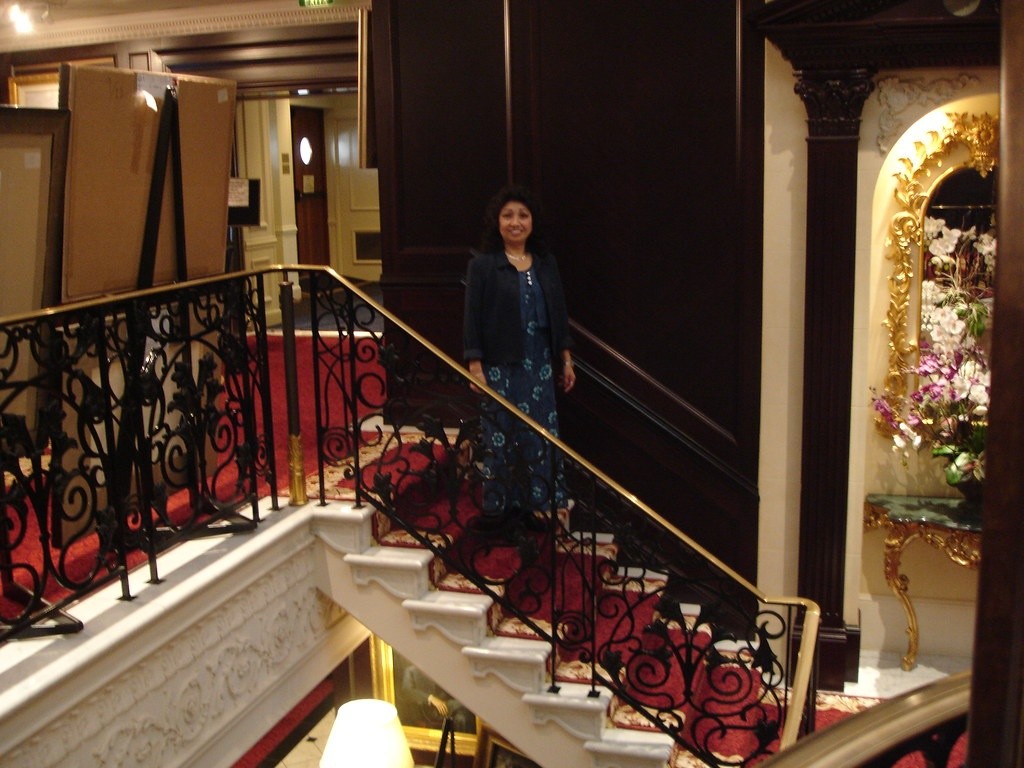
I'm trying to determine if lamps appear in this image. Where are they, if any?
[319,699,415,768]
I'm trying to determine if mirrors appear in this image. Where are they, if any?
[914,163,1000,422]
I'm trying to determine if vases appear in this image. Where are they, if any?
[948,420,988,516]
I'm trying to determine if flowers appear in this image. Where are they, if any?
[873,294,990,486]
[922,216,997,362]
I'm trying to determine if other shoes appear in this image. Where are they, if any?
[482,517,513,541]
[514,506,547,533]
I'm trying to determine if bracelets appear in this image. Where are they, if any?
[563,359,573,369]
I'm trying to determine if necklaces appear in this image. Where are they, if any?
[505,251,528,259]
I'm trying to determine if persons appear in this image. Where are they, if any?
[464,185,576,533]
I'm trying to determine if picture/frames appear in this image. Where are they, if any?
[369,632,481,756]
[473,724,545,768]
